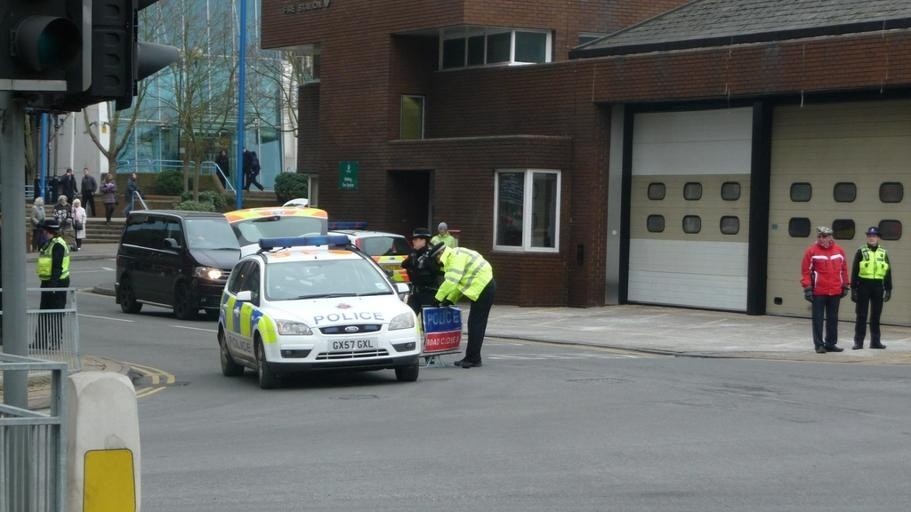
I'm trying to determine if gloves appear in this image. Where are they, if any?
[883,289,891,302]
[431,297,441,307]
[852,288,857,302]
[841,285,849,298]
[442,299,450,307]
[804,288,814,302]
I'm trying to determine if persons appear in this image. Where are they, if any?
[242,151,264,191]
[31,168,141,252]
[242,146,248,190]
[402,227,444,317]
[850,226,892,350]
[427,241,496,368]
[28,219,70,350]
[215,149,230,189]
[800,226,850,353]
[430,221,455,248]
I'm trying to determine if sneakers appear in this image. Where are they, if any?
[455,358,481,368]
[817,345,886,353]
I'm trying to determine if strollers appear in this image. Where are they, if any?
[57,216,79,252]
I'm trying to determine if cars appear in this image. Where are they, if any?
[329,227,416,298]
[213,206,426,386]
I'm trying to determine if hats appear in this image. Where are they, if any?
[817,225,833,235]
[429,241,445,257]
[411,227,432,238]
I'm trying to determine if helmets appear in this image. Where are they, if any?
[866,226,880,235]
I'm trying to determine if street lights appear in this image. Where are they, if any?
[174,45,204,202]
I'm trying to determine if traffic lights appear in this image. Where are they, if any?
[0,0,89,99]
[92,0,183,110]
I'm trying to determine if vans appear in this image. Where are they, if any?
[114,209,241,318]
